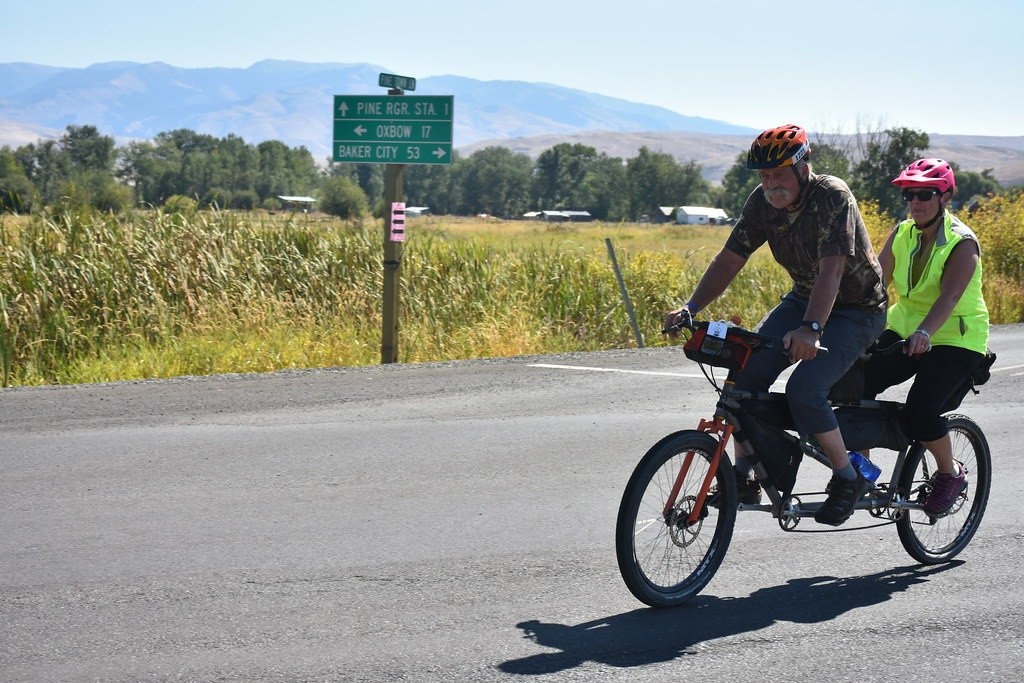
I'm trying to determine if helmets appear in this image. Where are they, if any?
[892,158,955,199]
[747,124,809,169]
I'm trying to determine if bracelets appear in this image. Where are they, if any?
[684,302,698,315]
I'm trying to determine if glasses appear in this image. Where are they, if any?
[901,190,937,201]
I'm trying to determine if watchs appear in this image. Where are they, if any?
[802,321,824,337]
[914,329,930,338]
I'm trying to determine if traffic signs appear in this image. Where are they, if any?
[333,94,454,164]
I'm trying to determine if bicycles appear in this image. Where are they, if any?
[615,310,996,608]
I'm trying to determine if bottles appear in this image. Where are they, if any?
[724,315,741,327]
[846,452,882,482]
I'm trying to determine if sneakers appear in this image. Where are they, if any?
[707,478,762,505]
[925,461,968,514]
[814,464,867,524]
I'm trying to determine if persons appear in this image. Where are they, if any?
[825,159,990,516]
[664,125,889,526]
